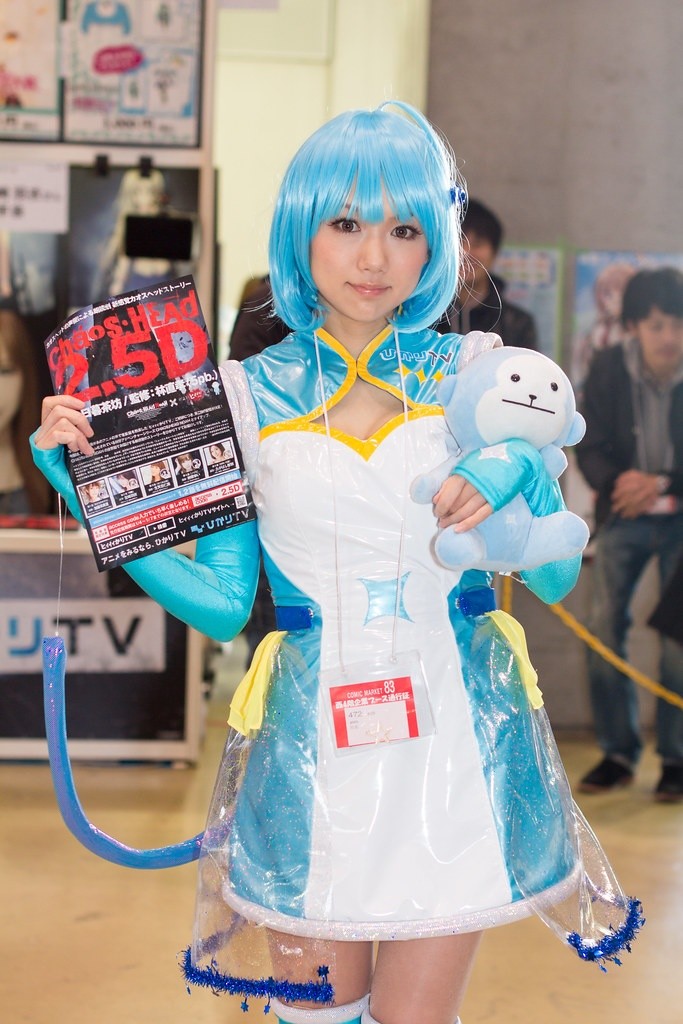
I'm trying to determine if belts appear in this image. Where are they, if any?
[274,587,499,631]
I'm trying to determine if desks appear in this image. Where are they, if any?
[0,527,206,772]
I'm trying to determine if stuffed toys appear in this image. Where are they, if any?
[409,330,588,605]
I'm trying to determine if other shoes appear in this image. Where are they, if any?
[656,765,683,802]
[580,756,635,792]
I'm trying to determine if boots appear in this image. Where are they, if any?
[271,992,369,1024]
[360,1009,461,1024]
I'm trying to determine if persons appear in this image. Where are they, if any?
[0,100,682,1023]
